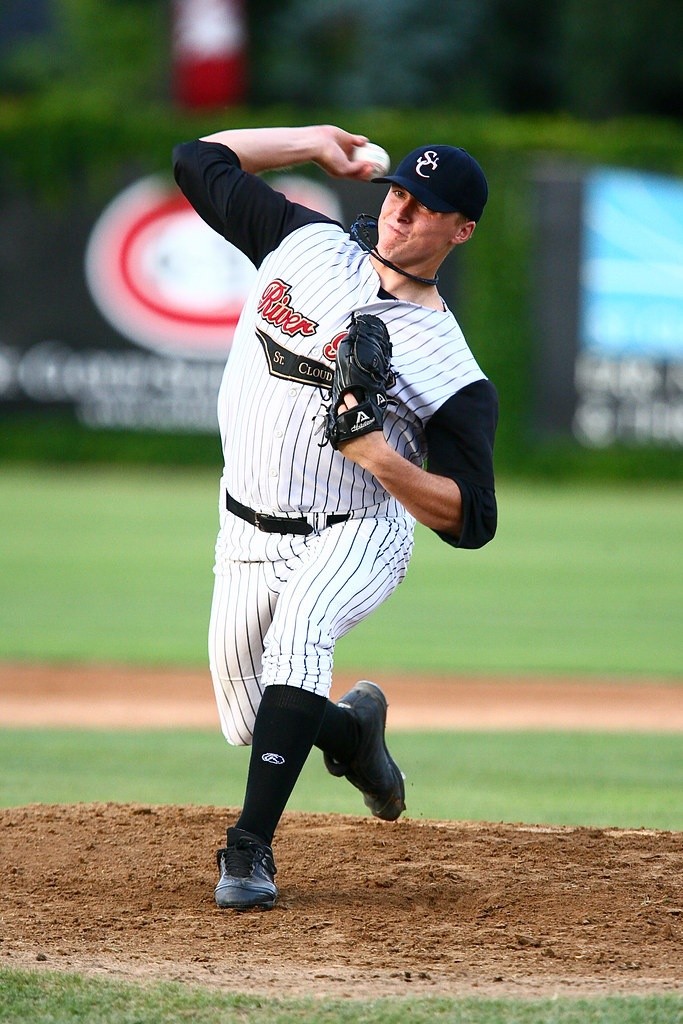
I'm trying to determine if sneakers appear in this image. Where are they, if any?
[323,681,407,821]
[215,827,277,908]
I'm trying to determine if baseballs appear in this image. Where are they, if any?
[357,142,390,179]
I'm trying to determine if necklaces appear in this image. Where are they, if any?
[352,213,439,284]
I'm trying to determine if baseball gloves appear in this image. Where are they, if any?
[326,307,395,452]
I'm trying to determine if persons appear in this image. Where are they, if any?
[172,123,499,913]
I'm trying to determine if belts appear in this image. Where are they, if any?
[224,490,352,535]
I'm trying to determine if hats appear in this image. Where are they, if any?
[369,145,488,224]
